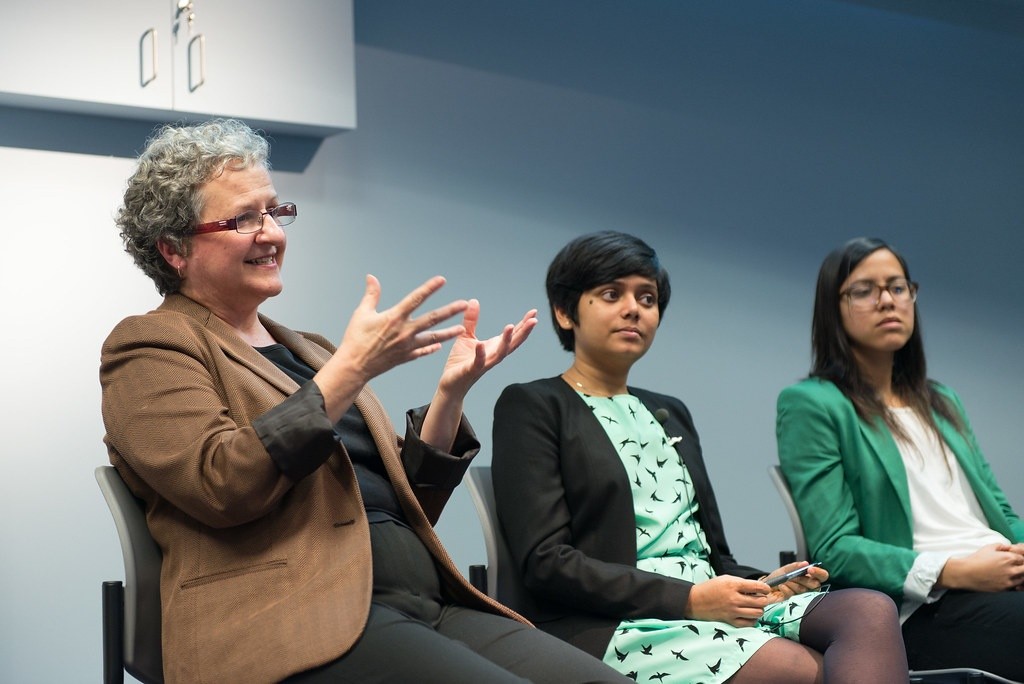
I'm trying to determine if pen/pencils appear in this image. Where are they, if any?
[744,562,822,597]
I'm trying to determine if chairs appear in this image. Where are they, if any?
[93,464,164,684]
[464,465,505,606]
[767,464,1023,684]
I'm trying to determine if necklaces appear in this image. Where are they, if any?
[564,373,613,397]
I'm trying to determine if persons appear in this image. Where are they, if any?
[490,231,908,683]
[98,119,638,682]
[776,239,1024,684]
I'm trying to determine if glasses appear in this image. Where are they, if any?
[182,203,297,234]
[839,282,919,306]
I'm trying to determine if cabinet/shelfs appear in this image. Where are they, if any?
[1,1,358,139]
